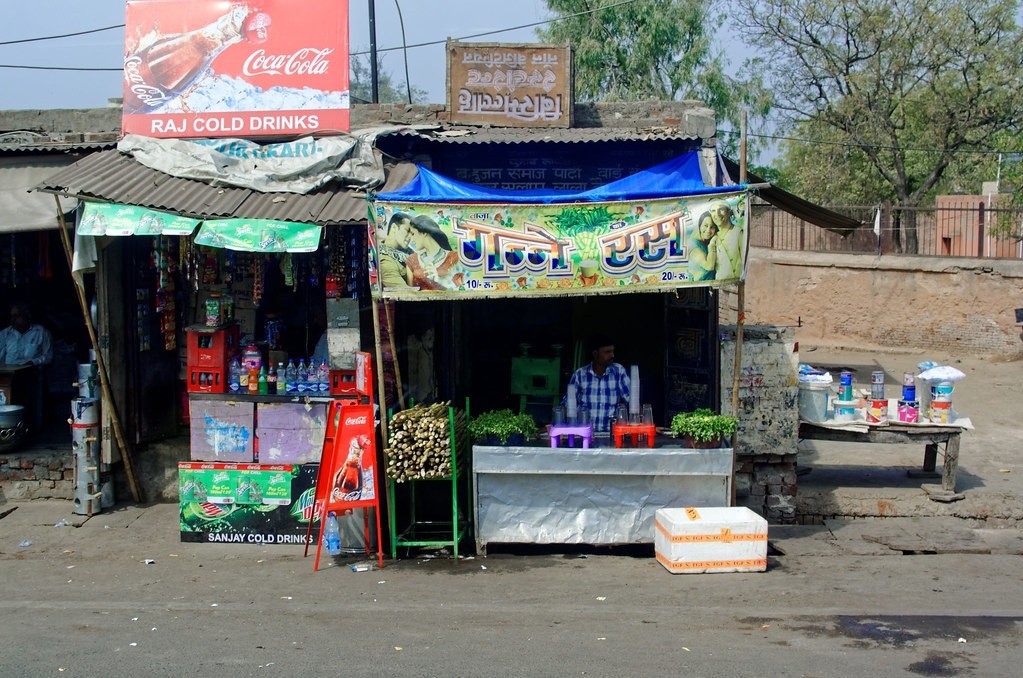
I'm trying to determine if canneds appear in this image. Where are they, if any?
[902,372,916,401]
[839,372,852,401]
[871,370,885,399]
[198,228,216,244]
[136,212,157,234]
[236,476,249,502]
[261,229,275,250]
[182,474,194,500]
[79,209,97,233]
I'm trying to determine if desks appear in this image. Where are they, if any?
[0,363,33,406]
[799,398,975,492]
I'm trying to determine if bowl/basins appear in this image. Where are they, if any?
[0,405,32,453]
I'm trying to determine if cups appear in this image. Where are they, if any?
[551,383,590,427]
[838,370,916,400]
[616,365,654,427]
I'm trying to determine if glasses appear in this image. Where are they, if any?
[8,313,22,319]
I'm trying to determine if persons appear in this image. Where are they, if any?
[406,214,458,290]
[378,212,419,290]
[706,197,744,280]
[686,211,719,281]
[557,341,644,431]
[0,302,53,434]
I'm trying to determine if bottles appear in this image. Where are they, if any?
[192,335,241,392]
[334,374,355,389]
[239,342,330,397]
[124,9,246,114]
[203,292,236,328]
[325,514,340,555]
[334,436,363,504]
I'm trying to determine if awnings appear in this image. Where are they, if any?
[719,154,866,239]
[26,147,421,253]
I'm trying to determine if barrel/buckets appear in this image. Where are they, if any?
[832,399,859,421]
[898,400,919,423]
[799,385,829,422]
[921,379,954,424]
[68,363,115,515]
[865,399,888,422]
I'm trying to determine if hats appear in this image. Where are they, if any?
[708,198,731,210]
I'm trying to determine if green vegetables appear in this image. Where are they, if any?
[470,408,539,445]
[543,206,627,238]
[671,408,740,444]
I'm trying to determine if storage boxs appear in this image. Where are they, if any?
[186,324,240,394]
[654,506,769,574]
[329,369,360,396]
